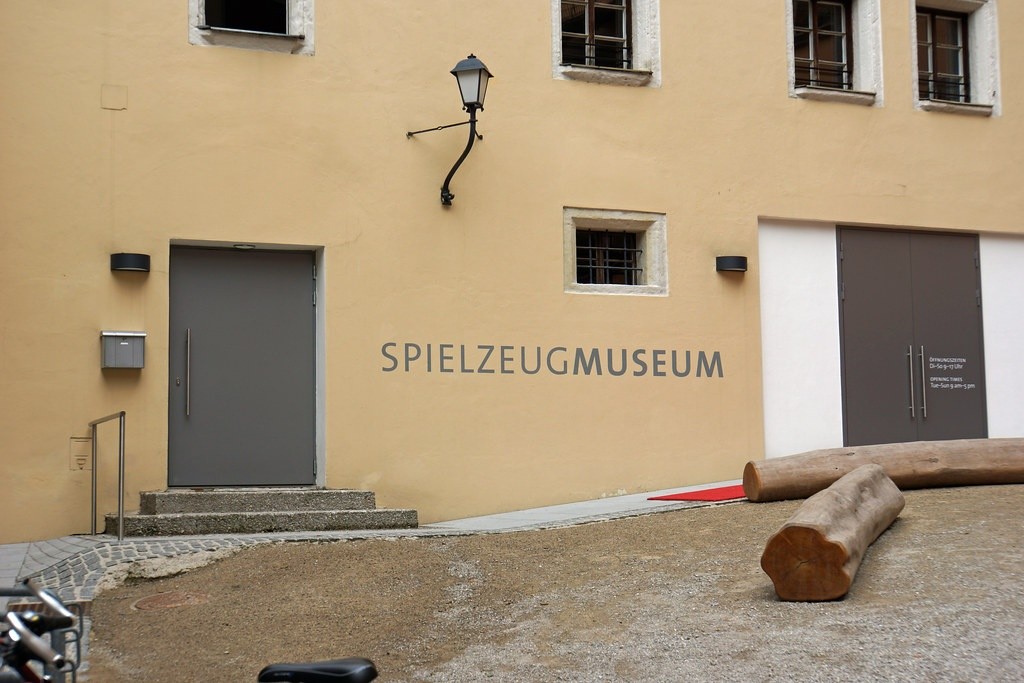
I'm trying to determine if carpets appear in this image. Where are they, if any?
[647,484,747,501]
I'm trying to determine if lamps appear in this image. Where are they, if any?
[715,256,748,272]
[110,252,152,272]
[441,53,495,206]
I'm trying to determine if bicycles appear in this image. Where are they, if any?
[0,576,377,683]
